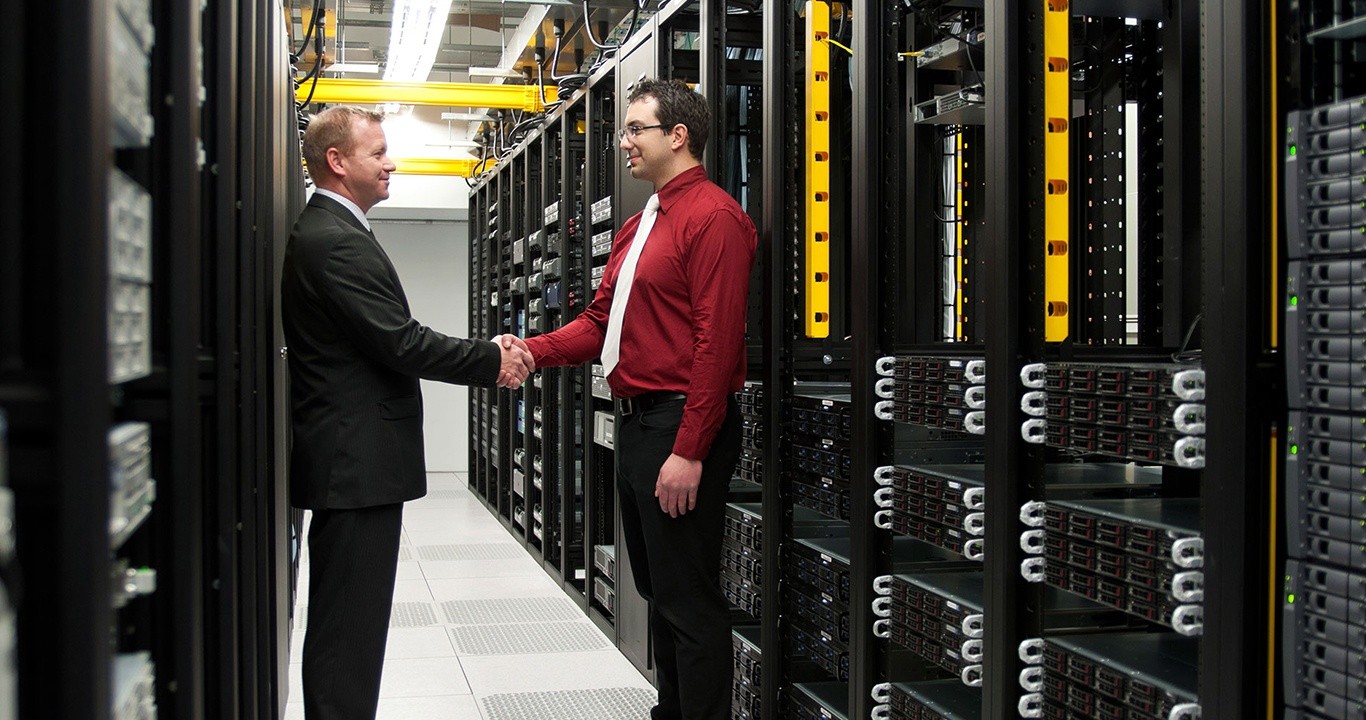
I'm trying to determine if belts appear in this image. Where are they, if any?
[619,391,687,416]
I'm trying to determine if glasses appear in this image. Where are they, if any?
[618,126,668,141]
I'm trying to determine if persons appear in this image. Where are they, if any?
[496,78,758,720]
[281,107,535,720]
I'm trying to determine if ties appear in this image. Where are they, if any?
[600,191,660,377]
